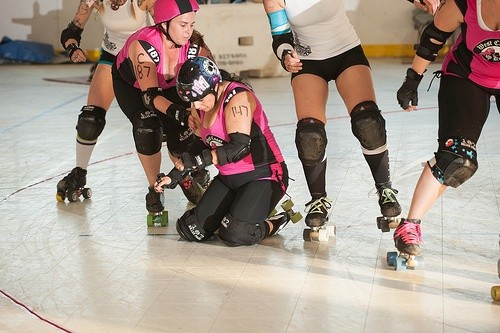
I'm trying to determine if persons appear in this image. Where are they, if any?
[263,0,441,227]
[393,0,500,279]
[57,0,157,191]
[153,56,292,247]
[110,0,217,214]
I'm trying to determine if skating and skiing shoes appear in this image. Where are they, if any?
[56,167,92,202]
[375,182,402,233]
[145,184,169,228]
[303,195,337,242]
[386,218,422,272]
[269,199,303,237]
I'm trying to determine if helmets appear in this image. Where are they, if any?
[176,56,222,102]
[153,0,199,24]
